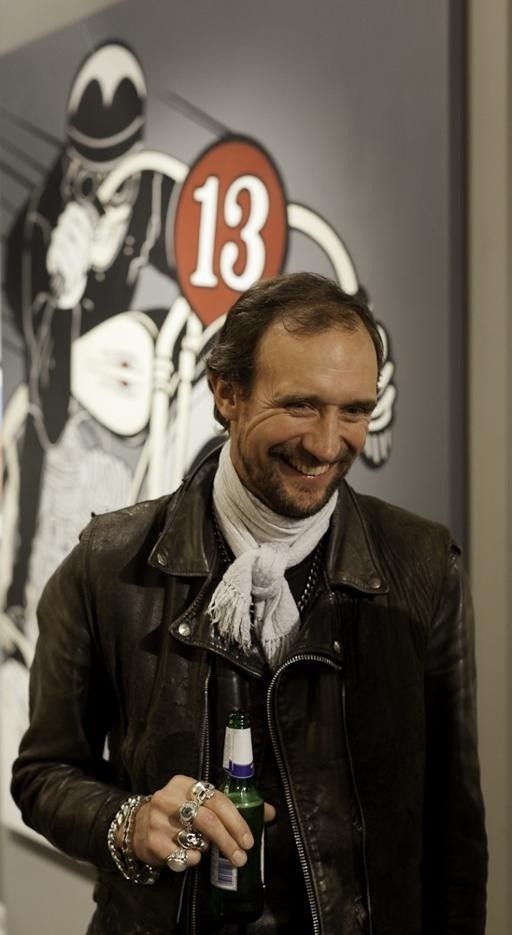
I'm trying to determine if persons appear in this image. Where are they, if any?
[8,268,492,933]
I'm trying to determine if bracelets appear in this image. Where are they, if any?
[104,795,142,887]
[120,794,162,885]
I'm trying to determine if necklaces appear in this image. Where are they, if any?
[211,506,323,632]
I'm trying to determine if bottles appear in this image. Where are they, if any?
[205,712,267,926]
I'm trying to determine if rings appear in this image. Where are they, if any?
[179,800,199,827]
[165,847,189,874]
[176,827,206,850]
[190,780,216,807]
